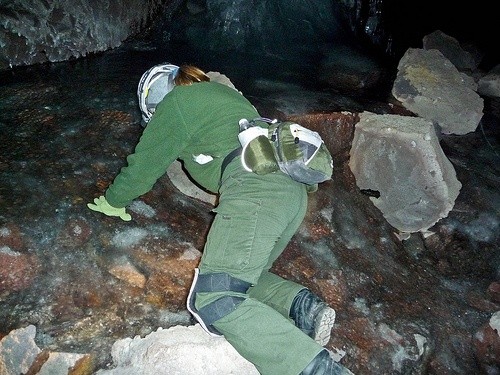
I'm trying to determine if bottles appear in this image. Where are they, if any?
[239,118,254,132]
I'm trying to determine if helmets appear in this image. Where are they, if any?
[137,63,180,125]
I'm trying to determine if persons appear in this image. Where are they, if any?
[85,62,359,375]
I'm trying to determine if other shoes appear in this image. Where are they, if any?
[289,288,336,347]
[299,348,354,375]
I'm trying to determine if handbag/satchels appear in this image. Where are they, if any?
[268,121,333,184]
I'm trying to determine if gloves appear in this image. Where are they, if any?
[87,195,131,221]
